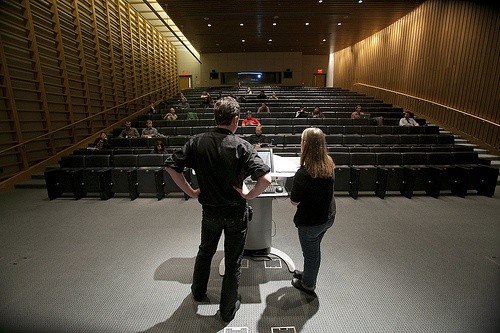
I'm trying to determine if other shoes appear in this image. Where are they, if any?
[221,294,241,323]
[292,277,314,295]
[294,270,304,280]
[191,284,207,302]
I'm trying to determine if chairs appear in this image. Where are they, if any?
[44,85,500,200]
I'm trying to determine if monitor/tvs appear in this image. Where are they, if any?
[256,149,274,173]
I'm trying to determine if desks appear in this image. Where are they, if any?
[218,183,295,276]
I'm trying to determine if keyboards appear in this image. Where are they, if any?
[246,184,275,193]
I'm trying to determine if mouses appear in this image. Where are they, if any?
[276,186,283,192]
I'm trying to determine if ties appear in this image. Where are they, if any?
[407,119,411,124]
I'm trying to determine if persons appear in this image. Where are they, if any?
[146,102,160,115]
[257,103,270,113]
[295,105,325,118]
[94,133,112,149]
[289,128,337,294]
[231,82,278,102]
[164,108,178,119]
[242,110,261,126]
[248,126,268,148]
[164,96,273,323]
[398,111,419,128]
[178,90,222,107]
[120,120,168,152]
[350,105,366,118]
